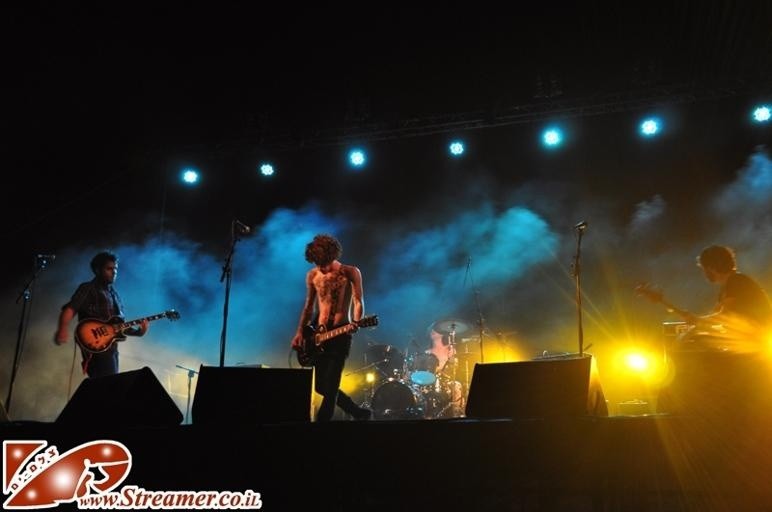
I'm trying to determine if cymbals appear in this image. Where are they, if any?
[453,352,478,362]
[433,318,474,337]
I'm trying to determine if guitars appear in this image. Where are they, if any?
[296,312,380,368]
[75,308,181,355]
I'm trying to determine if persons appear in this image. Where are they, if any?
[680,244,772,378]
[53,251,148,379]
[422,323,466,419]
[291,233,373,423]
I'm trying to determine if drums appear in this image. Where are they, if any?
[370,378,427,421]
[410,351,439,385]
[362,344,405,387]
[429,380,466,417]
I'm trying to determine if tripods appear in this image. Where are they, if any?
[435,326,465,419]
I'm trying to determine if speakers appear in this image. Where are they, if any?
[465,355,608,417]
[55,366,184,424]
[656,348,772,415]
[191,364,316,426]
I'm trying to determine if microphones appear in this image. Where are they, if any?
[236,219,251,235]
[574,221,588,230]
[38,253,55,261]
